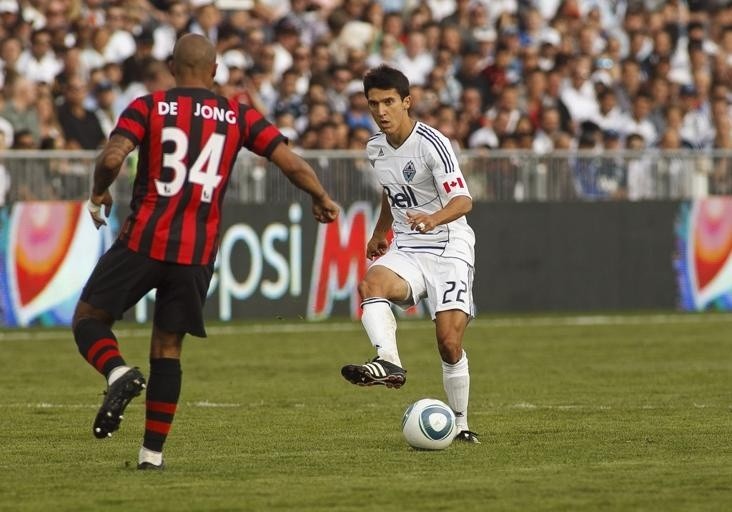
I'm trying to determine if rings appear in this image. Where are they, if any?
[419,224,426,230]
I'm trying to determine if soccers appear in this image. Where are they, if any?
[401,398,457,450]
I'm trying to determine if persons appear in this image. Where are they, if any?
[72,34,339,470]
[341,65,482,446]
[2,1,732,206]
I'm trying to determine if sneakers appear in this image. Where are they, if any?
[136,458,172,473]
[456,431,482,446]
[341,357,408,388]
[93,366,146,438]
